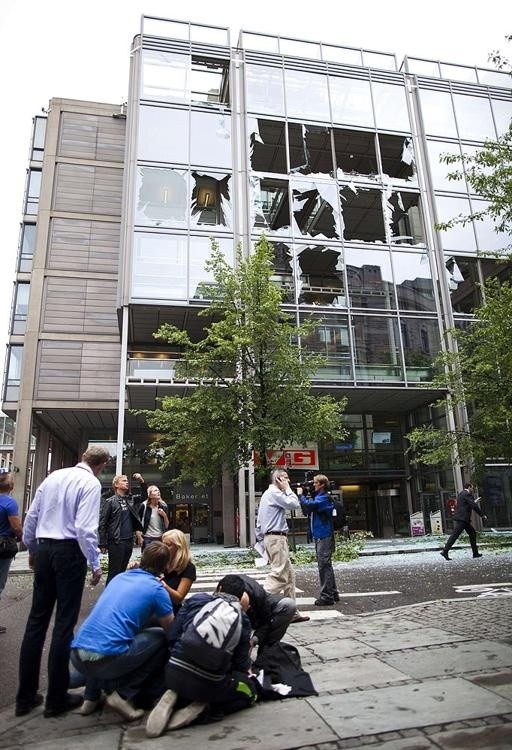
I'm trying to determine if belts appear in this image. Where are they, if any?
[35,537,53,544]
[266,531,286,536]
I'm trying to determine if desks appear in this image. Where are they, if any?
[192,526,208,542]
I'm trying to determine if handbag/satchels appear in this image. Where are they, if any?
[333,499,346,528]
[0,536,19,558]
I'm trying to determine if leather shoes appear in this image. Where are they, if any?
[291,616,310,623]
[15,694,44,716]
[440,551,451,560]
[43,693,84,718]
[473,553,482,558]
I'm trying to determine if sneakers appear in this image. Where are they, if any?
[146,688,178,738]
[107,691,145,721]
[82,694,105,715]
[0,626,7,633]
[166,699,207,731]
[314,594,339,606]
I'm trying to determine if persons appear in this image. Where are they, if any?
[70,528,296,740]
[254,468,311,623]
[440,482,488,560]
[135,484,169,554]
[15,444,111,717]
[297,474,340,606]
[98,472,148,590]
[0,472,23,634]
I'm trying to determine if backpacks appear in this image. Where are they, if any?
[180,593,244,673]
